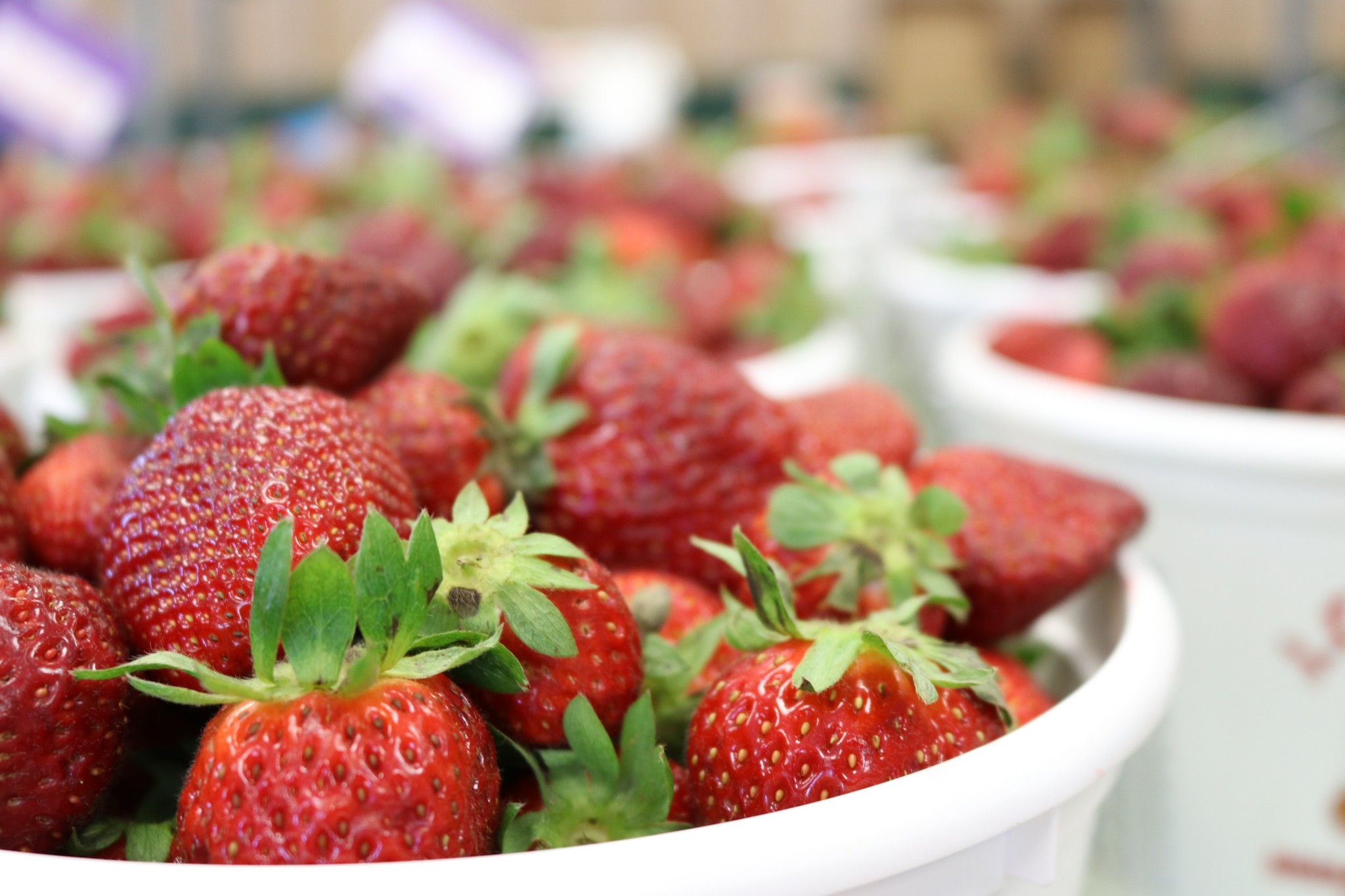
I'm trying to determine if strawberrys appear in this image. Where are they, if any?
[0,87,1345,865]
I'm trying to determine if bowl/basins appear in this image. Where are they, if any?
[0,128,1345,896]
